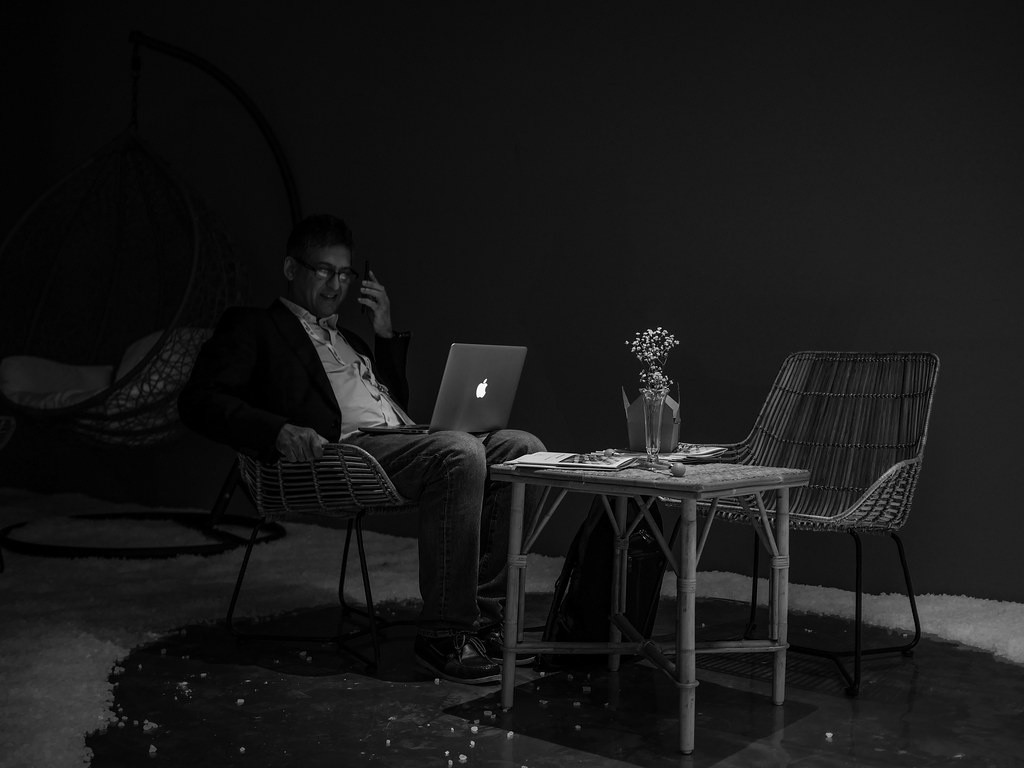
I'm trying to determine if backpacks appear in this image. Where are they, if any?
[534,494,668,671]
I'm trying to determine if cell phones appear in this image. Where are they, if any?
[361,260,377,318]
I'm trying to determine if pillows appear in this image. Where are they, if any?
[0,327,215,447]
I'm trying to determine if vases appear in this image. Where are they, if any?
[639,391,666,467]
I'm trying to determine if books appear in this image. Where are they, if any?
[503,446,728,472]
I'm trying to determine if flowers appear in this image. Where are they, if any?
[626,328,682,456]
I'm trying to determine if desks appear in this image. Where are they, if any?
[490,462,812,756]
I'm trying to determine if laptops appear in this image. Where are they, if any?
[359,343,527,436]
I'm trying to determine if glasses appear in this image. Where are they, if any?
[294,258,361,285]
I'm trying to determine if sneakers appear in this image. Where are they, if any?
[474,624,536,665]
[411,631,503,684]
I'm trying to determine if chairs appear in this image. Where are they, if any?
[224,439,420,672]
[638,351,943,697]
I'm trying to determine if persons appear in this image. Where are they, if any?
[178,213,547,685]
